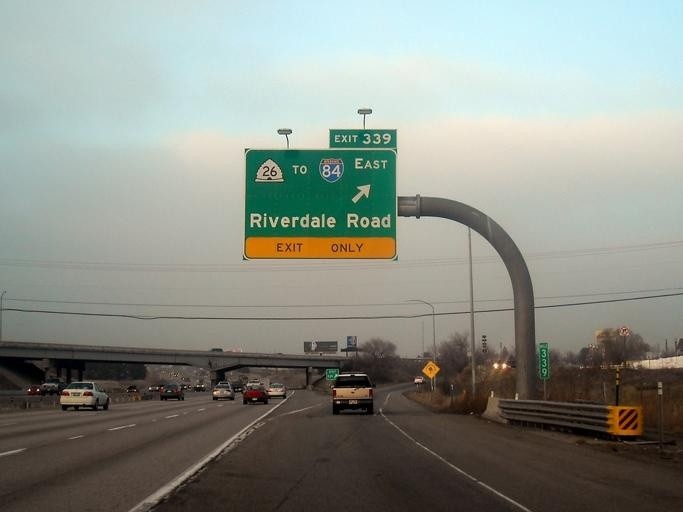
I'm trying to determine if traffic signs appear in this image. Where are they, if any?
[242,129,397,260]
[325,369,339,380]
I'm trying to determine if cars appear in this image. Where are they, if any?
[125,379,286,404]
[26,378,109,410]
[414,375,424,384]
[328,372,375,414]
[210,348,222,352]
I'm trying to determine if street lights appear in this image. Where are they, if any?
[399,300,437,392]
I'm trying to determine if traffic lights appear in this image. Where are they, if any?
[481,335,487,352]
